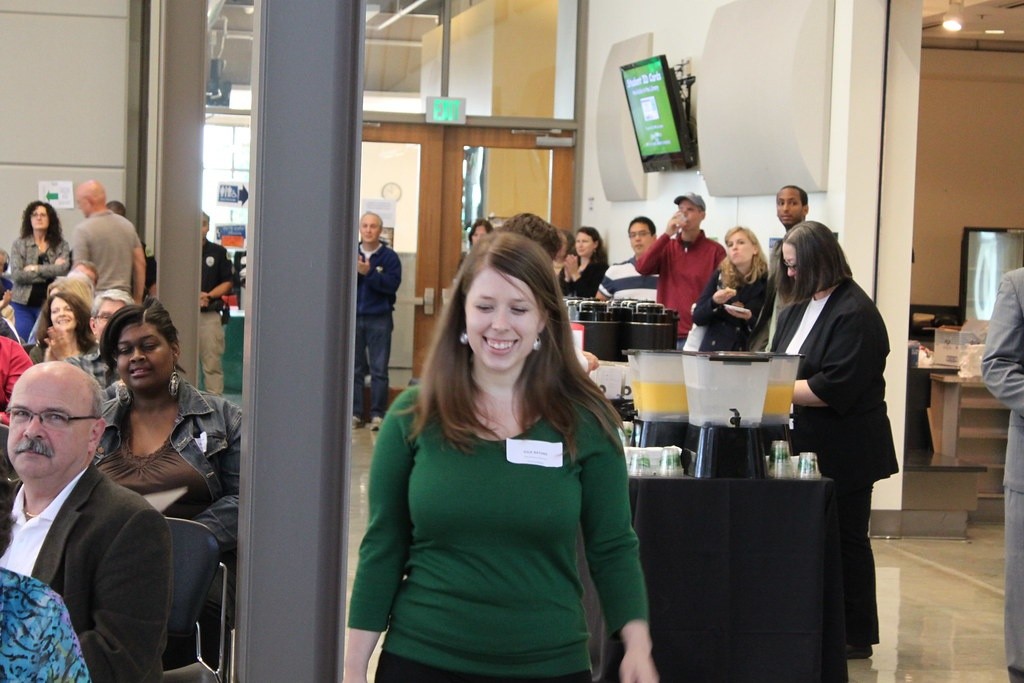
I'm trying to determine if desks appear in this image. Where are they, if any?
[909,365,957,381]
[576,469,848,683]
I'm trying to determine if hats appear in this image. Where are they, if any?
[674,192,706,211]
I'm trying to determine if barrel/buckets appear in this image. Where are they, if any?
[562,295,681,363]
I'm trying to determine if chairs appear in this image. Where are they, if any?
[164,517,229,683]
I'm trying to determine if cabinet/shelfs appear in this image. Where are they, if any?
[926,372,1013,524]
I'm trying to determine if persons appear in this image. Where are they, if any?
[981,266,1024,683]
[770,221,899,660]
[0,361,172,683]
[0,466,92,683]
[766,185,808,317]
[92,294,243,683]
[342,231,659,683]
[552,228,575,276]
[692,227,769,352]
[595,217,660,303]
[559,227,610,298]
[636,194,727,350]
[197,212,234,396]
[0,180,157,426]
[493,212,561,260]
[352,213,401,430]
[469,219,493,246]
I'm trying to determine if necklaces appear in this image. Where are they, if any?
[23,508,38,518]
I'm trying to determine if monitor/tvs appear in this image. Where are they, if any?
[620,55,698,172]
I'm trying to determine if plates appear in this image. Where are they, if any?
[724,303,750,314]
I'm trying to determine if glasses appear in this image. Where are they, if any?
[5,405,99,430]
[629,230,651,238]
[782,261,797,271]
[94,311,111,324]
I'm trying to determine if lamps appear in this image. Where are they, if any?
[942,0,965,29]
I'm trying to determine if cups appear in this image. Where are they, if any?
[765,439,822,481]
[673,213,688,232]
[628,447,684,478]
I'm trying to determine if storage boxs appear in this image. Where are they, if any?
[909,343,919,366]
[923,319,989,368]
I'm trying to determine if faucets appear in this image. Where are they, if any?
[728,407,742,429]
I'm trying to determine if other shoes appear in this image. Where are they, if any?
[370,416,382,430]
[351,415,365,429]
[846,644,873,659]
